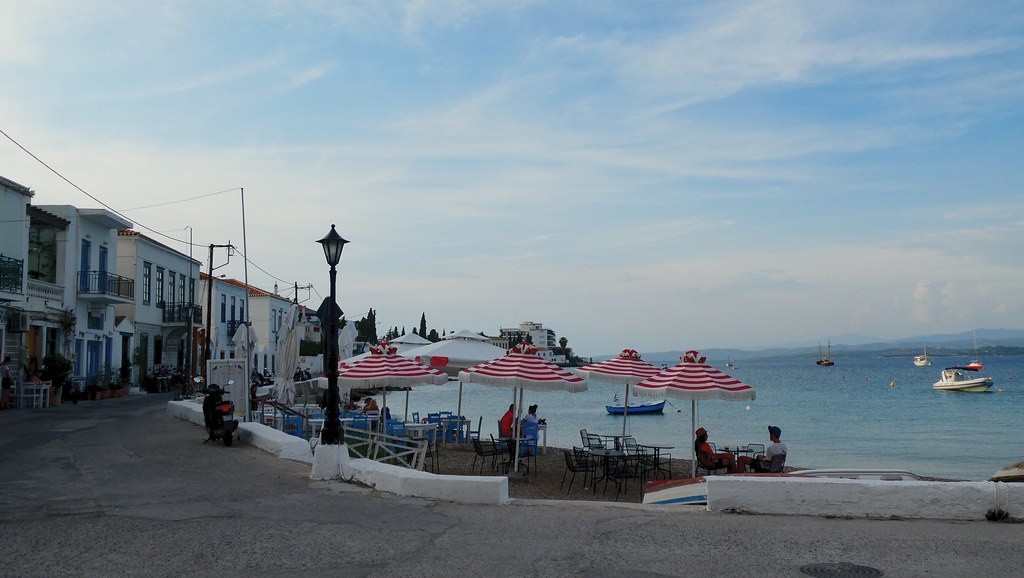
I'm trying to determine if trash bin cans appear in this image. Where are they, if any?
[194,376,204,391]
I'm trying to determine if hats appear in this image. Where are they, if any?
[696,427,707,438]
[768,425,781,437]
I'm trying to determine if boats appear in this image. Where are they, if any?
[931,369,994,392]
[913,340,932,367]
[726,355,734,367]
[605,398,669,415]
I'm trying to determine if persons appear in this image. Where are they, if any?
[25,369,55,393]
[524,406,540,444]
[501,404,518,437]
[695,427,737,473]
[738,426,787,472]
[348,400,357,410]
[363,397,378,415]
[0,356,12,410]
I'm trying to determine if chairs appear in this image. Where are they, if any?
[255,397,787,503]
[10,379,53,409]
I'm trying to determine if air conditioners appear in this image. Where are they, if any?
[11,310,30,333]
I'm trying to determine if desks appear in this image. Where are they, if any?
[601,434,632,451]
[306,417,353,442]
[441,419,471,445]
[494,438,534,474]
[587,450,626,494]
[396,422,437,454]
[527,424,548,454]
[638,443,674,481]
[717,448,754,462]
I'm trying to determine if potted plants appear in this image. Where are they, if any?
[40,354,74,406]
[76,368,126,401]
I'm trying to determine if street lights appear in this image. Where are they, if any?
[308,224,350,482]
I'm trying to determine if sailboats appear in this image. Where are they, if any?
[944,330,985,371]
[815,337,835,366]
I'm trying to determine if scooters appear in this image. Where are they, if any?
[193,375,241,448]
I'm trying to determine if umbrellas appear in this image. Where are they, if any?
[272,304,300,430]
[572,349,664,449]
[338,321,358,359]
[232,324,257,375]
[458,341,588,472]
[388,332,433,422]
[318,339,448,440]
[400,329,507,442]
[632,350,756,478]
